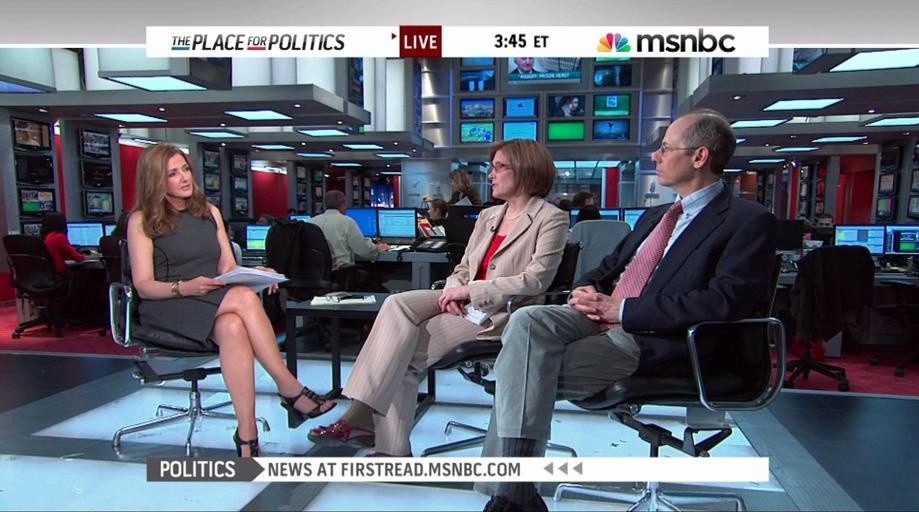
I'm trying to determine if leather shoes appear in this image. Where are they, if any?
[362,452,415,457]
[307,419,375,450]
[482,486,548,511]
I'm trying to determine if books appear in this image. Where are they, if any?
[464,301,506,326]
[211,267,290,295]
[310,295,377,306]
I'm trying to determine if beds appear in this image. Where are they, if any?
[286,292,436,429]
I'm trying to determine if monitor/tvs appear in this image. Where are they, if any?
[884,223,919,256]
[377,208,417,238]
[834,224,885,257]
[874,141,919,222]
[9,114,117,250]
[623,208,650,231]
[795,163,825,221]
[755,170,773,211]
[569,208,621,229]
[203,148,248,217]
[245,224,273,251]
[811,227,834,247]
[775,220,803,251]
[296,164,324,216]
[352,173,371,208]
[343,208,377,237]
[452,57,641,142]
[289,215,311,223]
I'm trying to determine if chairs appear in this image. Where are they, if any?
[773,246,870,391]
[100,236,126,337]
[870,279,919,377]
[2,234,82,339]
[421,243,585,456]
[109,238,270,458]
[553,252,787,512]
[267,221,361,352]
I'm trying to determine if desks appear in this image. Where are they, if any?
[777,270,919,357]
[65,256,452,289]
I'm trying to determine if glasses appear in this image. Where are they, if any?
[659,144,699,154]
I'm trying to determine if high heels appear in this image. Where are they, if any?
[281,386,337,424]
[232,427,263,458]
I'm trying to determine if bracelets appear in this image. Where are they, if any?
[171,280,183,297]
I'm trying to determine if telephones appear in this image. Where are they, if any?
[408,236,447,252]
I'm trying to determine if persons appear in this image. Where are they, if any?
[421,168,482,229]
[124,143,338,458]
[544,192,601,224]
[472,108,780,511]
[511,57,540,74]
[39,212,88,274]
[554,95,582,118]
[794,212,837,255]
[234,189,312,250]
[308,191,393,293]
[307,138,571,458]
[112,211,129,237]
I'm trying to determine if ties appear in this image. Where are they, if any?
[597,200,682,334]
